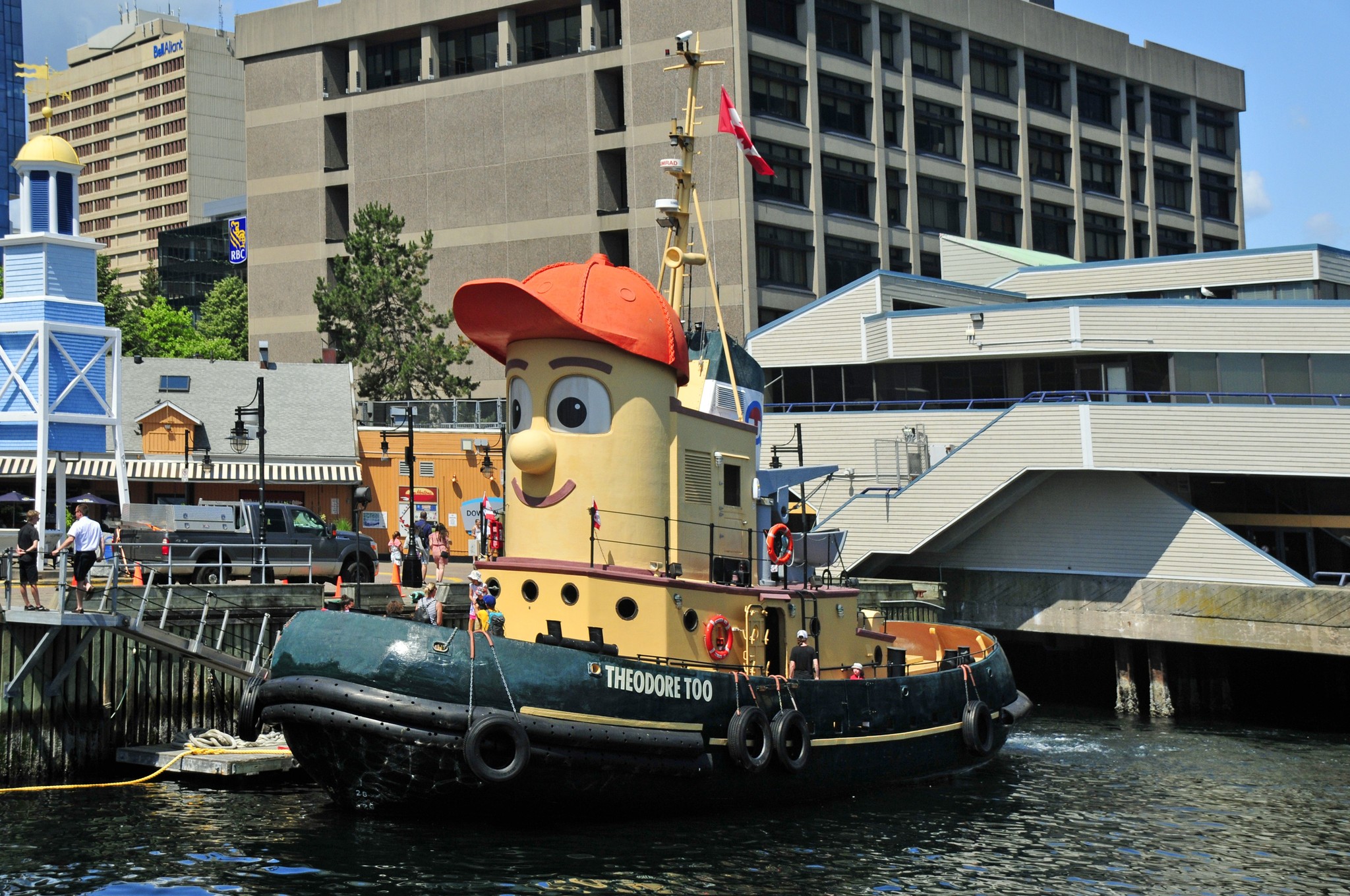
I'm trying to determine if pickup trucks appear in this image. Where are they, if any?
[111,497,379,585]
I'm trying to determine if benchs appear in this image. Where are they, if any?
[435,581,471,606]
[338,581,405,606]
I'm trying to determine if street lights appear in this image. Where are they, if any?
[351,487,372,609]
[380,409,422,589]
[481,427,506,511]
[225,377,275,585]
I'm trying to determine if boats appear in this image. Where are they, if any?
[236,27,1033,829]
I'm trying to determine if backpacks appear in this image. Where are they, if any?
[416,522,428,549]
[412,598,436,623]
[484,609,506,636]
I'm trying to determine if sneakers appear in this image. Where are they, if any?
[24,605,35,611]
[35,605,49,612]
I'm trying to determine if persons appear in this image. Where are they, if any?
[16,509,51,611]
[403,524,428,587]
[340,594,354,612]
[471,519,491,566]
[474,595,505,638]
[429,523,451,583]
[849,662,865,680]
[383,600,404,618]
[388,531,404,583]
[467,570,488,632]
[788,630,819,680]
[415,582,443,626]
[51,504,105,613]
[414,511,432,585]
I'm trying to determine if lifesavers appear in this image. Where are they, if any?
[727,705,770,768]
[704,615,733,660]
[235,677,266,742]
[463,713,530,780]
[766,523,794,565]
[963,699,995,754]
[770,709,811,768]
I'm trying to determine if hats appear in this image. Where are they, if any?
[482,594,496,606]
[851,662,862,670]
[467,570,482,582]
[797,630,808,639]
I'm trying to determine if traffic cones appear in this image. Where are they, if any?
[133,562,143,586]
[333,576,343,598]
[390,562,406,598]
[71,575,77,586]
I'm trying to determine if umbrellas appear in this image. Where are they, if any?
[66,492,118,506]
[0,490,35,528]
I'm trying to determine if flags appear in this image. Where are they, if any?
[482,495,495,523]
[719,87,777,175]
[592,500,602,530]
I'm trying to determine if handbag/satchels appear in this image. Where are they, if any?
[440,550,448,558]
[394,553,401,560]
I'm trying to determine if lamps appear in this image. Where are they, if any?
[668,562,683,580]
[843,467,855,477]
[649,561,663,577]
[656,216,680,240]
[970,312,985,322]
[788,603,796,618]
[837,604,844,619]
[165,424,171,432]
[134,430,142,436]
[808,574,823,591]
[673,593,683,610]
[714,451,725,468]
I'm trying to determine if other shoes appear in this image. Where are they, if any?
[71,608,83,612]
[422,581,426,586]
[85,585,95,600]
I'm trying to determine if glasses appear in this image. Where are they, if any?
[74,511,78,513]
[37,517,40,521]
